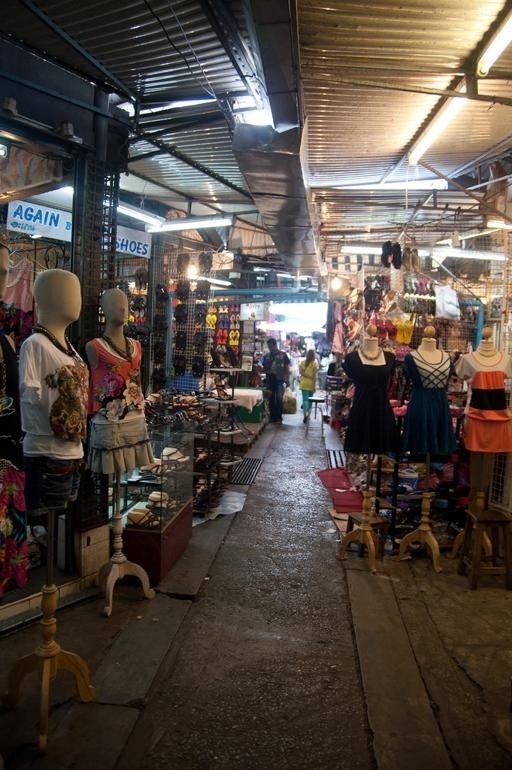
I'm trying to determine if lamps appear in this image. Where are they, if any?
[187,276,232,288]
[144,213,237,234]
[252,265,313,282]
[472,7,511,79]
[486,220,512,230]
[52,185,167,226]
[339,245,508,261]
[405,77,473,167]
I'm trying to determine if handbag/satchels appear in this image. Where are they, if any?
[282,388,297,414]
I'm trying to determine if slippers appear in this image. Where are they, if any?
[390,398,408,416]
[155,314,168,337]
[152,368,166,392]
[125,325,149,346]
[398,467,419,478]
[376,319,387,338]
[177,254,190,274]
[135,268,148,290]
[155,343,165,363]
[176,331,186,351]
[195,302,206,326]
[192,333,207,354]
[404,320,413,345]
[134,296,146,318]
[127,474,167,485]
[174,304,188,325]
[175,280,190,299]
[207,305,241,354]
[156,284,168,308]
[192,357,204,378]
[196,280,210,300]
[385,320,397,340]
[394,321,404,343]
[199,252,212,274]
[173,355,186,376]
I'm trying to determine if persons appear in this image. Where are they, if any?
[0,323,19,403]
[298,348,319,424]
[405,324,453,443]
[262,338,290,425]
[19,268,92,519]
[345,322,396,446]
[458,326,512,442]
[31,323,76,357]
[86,287,154,477]
[102,335,133,368]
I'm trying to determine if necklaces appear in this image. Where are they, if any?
[476,351,499,358]
[415,347,443,365]
[359,346,382,360]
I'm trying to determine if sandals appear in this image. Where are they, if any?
[193,475,226,510]
[195,446,222,472]
[216,446,241,462]
[125,508,160,529]
[160,449,190,464]
[187,407,208,421]
[218,389,236,401]
[146,492,184,512]
[174,395,207,408]
[202,423,239,432]
[138,465,176,477]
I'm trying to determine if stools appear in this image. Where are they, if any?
[346,511,389,557]
[305,397,325,439]
[456,508,512,592]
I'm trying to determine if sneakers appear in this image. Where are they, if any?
[364,287,373,311]
[491,296,506,317]
[403,247,412,271]
[303,416,307,422]
[372,287,381,309]
[412,249,420,272]
[381,241,392,267]
[404,276,435,296]
[404,297,435,314]
[393,242,401,269]
